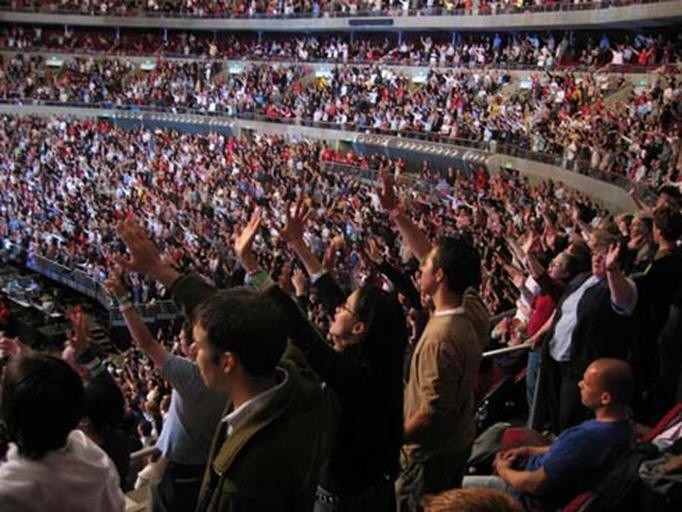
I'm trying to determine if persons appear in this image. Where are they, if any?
[0,0,682,512]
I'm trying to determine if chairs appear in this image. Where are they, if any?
[559,401,682,512]
